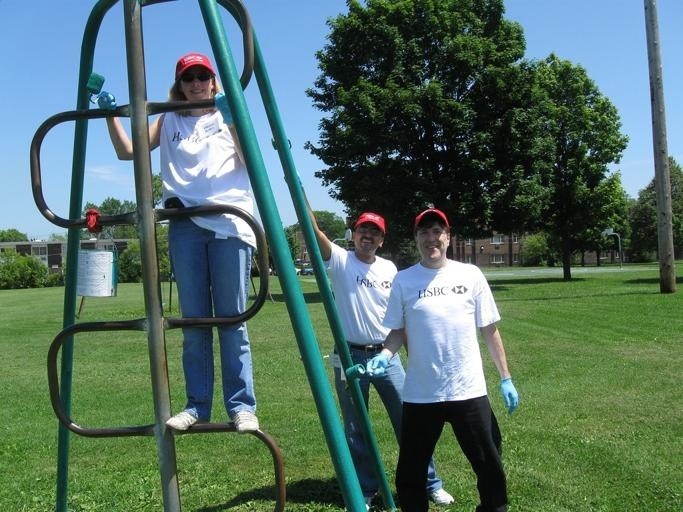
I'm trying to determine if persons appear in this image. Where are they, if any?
[363,207,522,511]
[95,52,264,434]
[293,174,457,512]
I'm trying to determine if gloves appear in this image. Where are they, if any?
[214,92,233,124]
[501,378,519,413]
[366,353,389,376]
[98,92,116,110]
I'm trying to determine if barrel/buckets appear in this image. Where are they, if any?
[76,239,118,299]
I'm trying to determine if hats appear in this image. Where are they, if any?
[175,54,216,75]
[416,209,449,228]
[355,212,386,235]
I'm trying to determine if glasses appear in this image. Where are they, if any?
[181,73,211,84]
[357,226,380,235]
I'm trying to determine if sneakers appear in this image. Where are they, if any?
[429,489,454,505]
[231,411,259,432]
[166,406,199,434]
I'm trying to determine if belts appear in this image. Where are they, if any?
[350,343,383,352]
